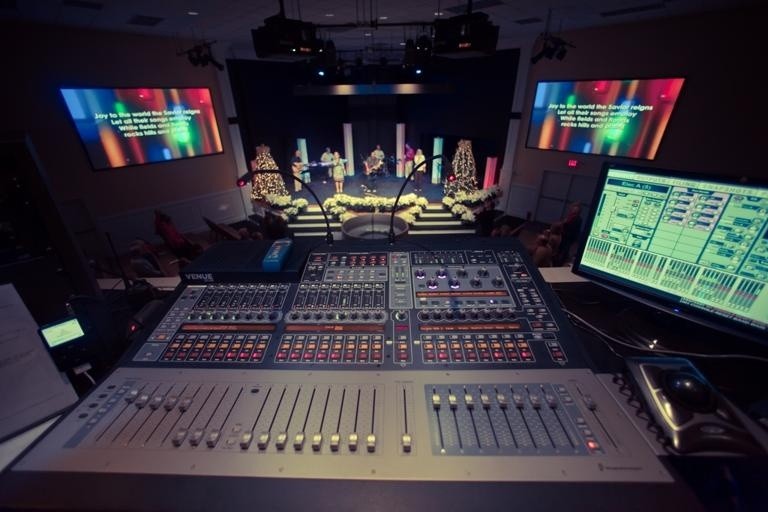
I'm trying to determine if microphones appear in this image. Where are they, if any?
[386,153,456,244]
[237,167,335,245]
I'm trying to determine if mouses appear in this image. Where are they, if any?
[660,369,719,414]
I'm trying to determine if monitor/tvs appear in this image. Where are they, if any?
[571,161,768,346]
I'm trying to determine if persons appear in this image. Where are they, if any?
[404,142,415,182]
[364,151,381,192]
[413,148,426,192]
[320,147,334,179]
[330,151,347,193]
[374,145,385,177]
[290,150,304,193]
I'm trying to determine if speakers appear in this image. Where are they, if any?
[250,14,318,61]
[432,11,500,60]
